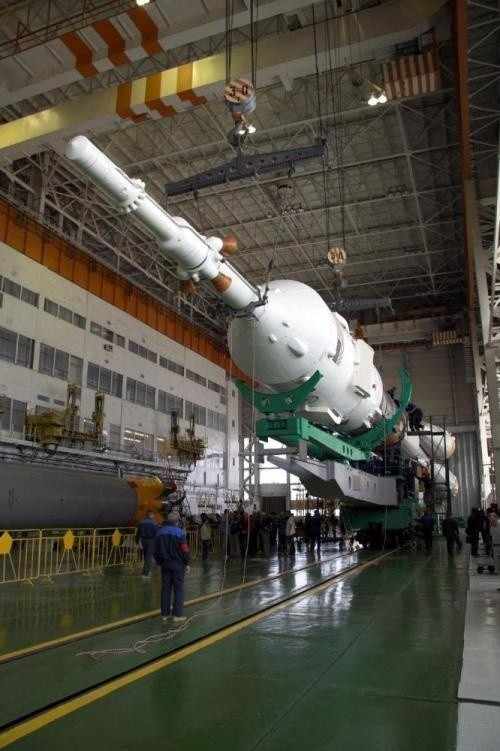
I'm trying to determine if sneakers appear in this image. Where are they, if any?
[162,616,169,621]
[143,574,150,578]
[173,616,187,623]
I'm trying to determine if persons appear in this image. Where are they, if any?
[487,513,500,591]
[134,509,161,579]
[152,511,192,623]
[387,386,397,400]
[465,503,500,557]
[414,510,435,554]
[405,407,419,432]
[415,468,433,508]
[408,402,425,431]
[440,512,460,561]
[199,503,339,561]
